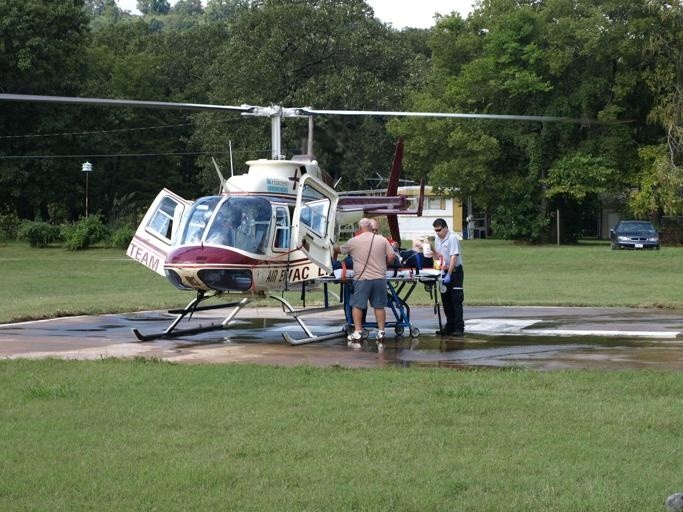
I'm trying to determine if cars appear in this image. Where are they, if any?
[609,220,661,250]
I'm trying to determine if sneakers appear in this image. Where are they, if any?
[375,331,385,340]
[347,331,362,341]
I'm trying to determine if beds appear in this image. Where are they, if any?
[331,268,438,338]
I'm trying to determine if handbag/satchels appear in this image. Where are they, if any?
[349,285,353,293]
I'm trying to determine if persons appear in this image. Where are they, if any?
[334,216,396,344]
[333,238,434,267]
[345,218,378,324]
[432,218,465,336]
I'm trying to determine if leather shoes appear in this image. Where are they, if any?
[435,329,450,335]
[452,331,462,337]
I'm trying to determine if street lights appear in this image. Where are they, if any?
[81,161,93,217]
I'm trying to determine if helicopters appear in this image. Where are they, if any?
[0,93,562,346]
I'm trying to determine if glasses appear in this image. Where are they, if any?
[435,228,442,232]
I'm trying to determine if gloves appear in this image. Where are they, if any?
[443,273,450,284]
